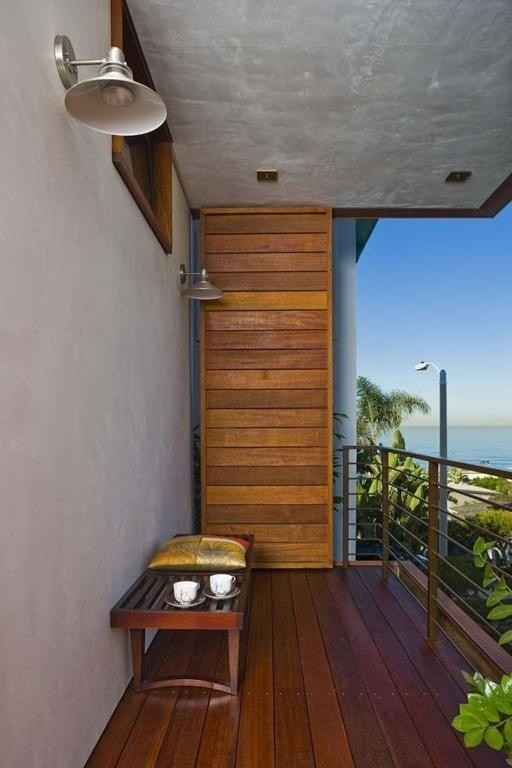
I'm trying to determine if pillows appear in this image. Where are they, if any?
[147,535,251,571]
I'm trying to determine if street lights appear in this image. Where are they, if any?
[415,356,450,563]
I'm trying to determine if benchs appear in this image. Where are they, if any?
[110,533,254,696]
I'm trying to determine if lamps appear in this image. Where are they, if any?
[180,263,223,300]
[53,35,168,138]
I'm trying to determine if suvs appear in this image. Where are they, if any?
[357,545,428,563]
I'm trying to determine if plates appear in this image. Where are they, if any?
[204,586,241,600]
[163,594,206,608]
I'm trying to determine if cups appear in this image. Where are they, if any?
[209,573,237,596]
[173,580,202,602]
